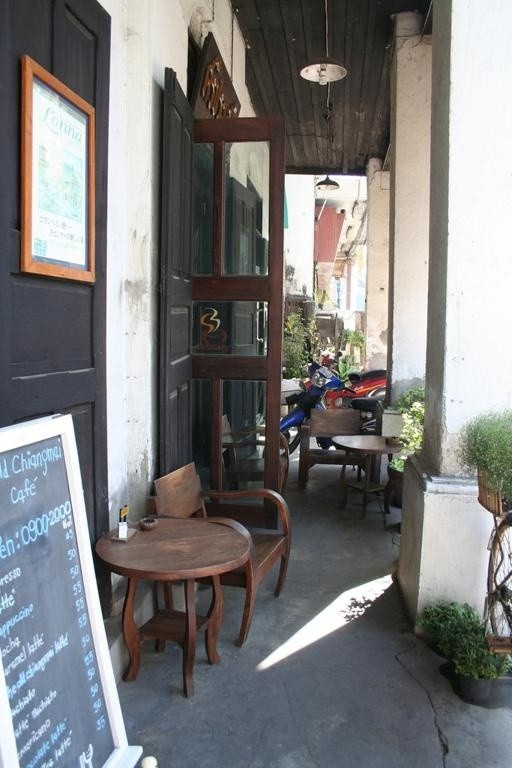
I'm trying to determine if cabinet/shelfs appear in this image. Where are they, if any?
[286,295,343,349]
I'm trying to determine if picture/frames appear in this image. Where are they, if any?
[22,56,99,283]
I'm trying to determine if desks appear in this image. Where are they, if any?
[95,518,252,697]
[332,435,402,518]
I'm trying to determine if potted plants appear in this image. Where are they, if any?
[456,409,512,517]
[452,635,512,708]
[388,456,407,508]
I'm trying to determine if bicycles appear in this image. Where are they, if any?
[483,510,512,638]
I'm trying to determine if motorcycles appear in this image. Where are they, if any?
[263,351,386,463]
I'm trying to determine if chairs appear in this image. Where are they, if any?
[149,462,291,647]
[299,408,361,489]
[222,415,289,496]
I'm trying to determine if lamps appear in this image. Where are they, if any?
[315,174,340,191]
[299,0,348,85]
